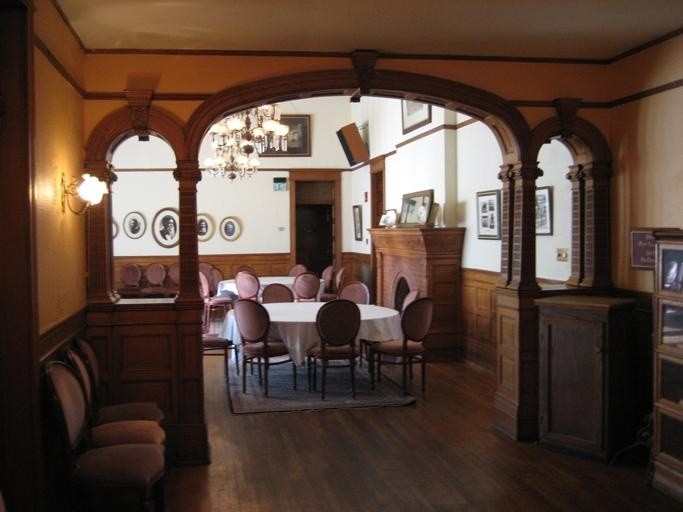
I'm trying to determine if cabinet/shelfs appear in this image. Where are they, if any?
[533,295,652,467]
[653,226,683,505]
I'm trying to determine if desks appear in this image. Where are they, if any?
[218,302,402,391]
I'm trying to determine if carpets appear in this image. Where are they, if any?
[223,355,417,413]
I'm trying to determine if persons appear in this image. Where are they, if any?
[224,220,234,236]
[128,217,140,234]
[197,218,208,236]
[157,213,177,243]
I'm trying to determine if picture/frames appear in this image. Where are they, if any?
[352,204,363,241]
[258,113,313,158]
[196,213,215,243]
[400,97,432,135]
[123,210,146,239]
[152,207,180,249]
[534,186,553,237]
[476,189,501,241]
[396,189,436,228]
[218,216,243,242]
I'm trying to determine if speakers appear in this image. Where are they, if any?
[336,123,369,166]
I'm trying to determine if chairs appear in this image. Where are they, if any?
[360,290,433,395]
[115,261,180,298]
[198,262,372,331]
[308,298,359,399]
[203,296,235,413]
[41,337,171,509]
[233,299,298,396]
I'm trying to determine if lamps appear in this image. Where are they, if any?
[56,166,106,217]
[200,103,277,182]
